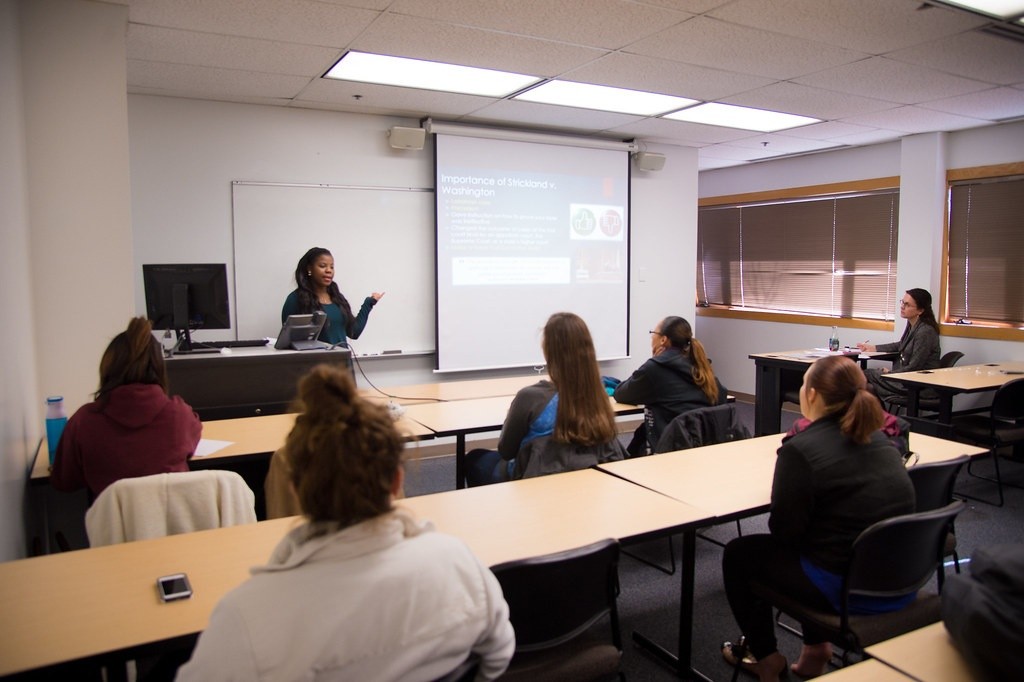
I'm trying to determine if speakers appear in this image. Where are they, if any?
[389,126,426,149]
[635,152,667,172]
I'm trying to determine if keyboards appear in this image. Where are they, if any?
[192,340,269,350]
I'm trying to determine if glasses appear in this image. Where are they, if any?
[900,300,918,309]
[649,331,662,337]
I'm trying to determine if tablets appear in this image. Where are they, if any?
[274,314,325,350]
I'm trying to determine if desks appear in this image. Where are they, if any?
[0,349,1024,682]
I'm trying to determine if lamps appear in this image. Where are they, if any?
[388,126,425,150]
[634,151,665,171]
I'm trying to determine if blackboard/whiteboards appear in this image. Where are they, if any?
[230,180,437,358]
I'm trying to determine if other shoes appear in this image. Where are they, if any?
[738,636,746,644]
[721,640,758,673]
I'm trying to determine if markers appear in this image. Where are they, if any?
[363,354,377,356]
[353,354,363,356]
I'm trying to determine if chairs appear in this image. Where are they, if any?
[888,351,964,416]
[487,538,622,682]
[908,454,970,573]
[620,404,750,575]
[86,468,256,548]
[262,444,304,521]
[951,377,1024,508]
[730,501,967,682]
[513,428,630,481]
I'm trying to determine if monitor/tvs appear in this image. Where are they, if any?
[142,264,230,354]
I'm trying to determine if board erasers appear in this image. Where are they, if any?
[380,350,402,354]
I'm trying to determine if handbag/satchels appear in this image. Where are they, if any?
[787,408,909,459]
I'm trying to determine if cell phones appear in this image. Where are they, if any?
[158,574,194,602]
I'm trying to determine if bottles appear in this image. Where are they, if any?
[829,326,839,351]
[43,396,69,465]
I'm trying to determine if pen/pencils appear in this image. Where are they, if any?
[862,339,869,345]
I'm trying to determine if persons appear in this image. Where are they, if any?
[53,317,203,497]
[613,316,728,459]
[722,355,916,682]
[175,364,516,682]
[858,288,951,416]
[465,312,618,488]
[282,247,385,349]
